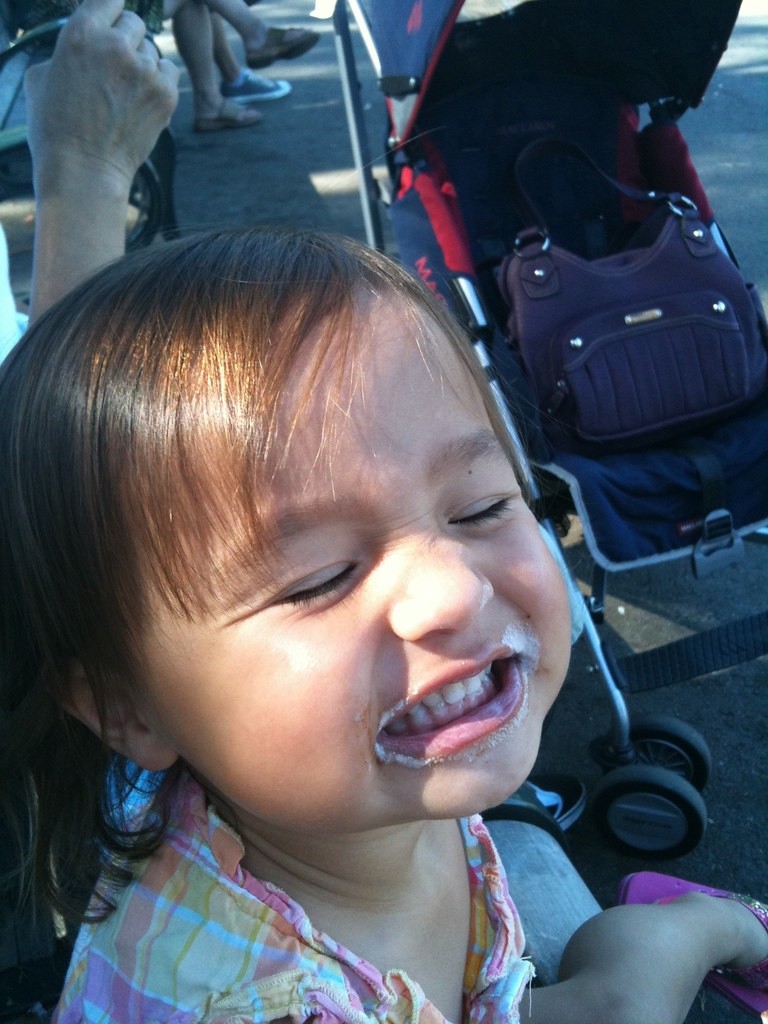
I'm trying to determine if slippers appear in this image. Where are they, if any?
[246,27,320,69]
[619,872,767,1018]
[194,97,264,132]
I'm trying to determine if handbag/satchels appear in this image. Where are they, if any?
[494,192,768,457]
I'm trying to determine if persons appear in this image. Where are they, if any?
[209,9,293,104]
[0,224,767,1024]
[117,0,320,133]
[0,1,181,368]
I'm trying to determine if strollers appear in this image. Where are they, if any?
[333,0,768,863]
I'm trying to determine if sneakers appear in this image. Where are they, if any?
[525,774,588,833]
[219,65,293,104]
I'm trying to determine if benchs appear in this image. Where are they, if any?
[0,0,185,259]
